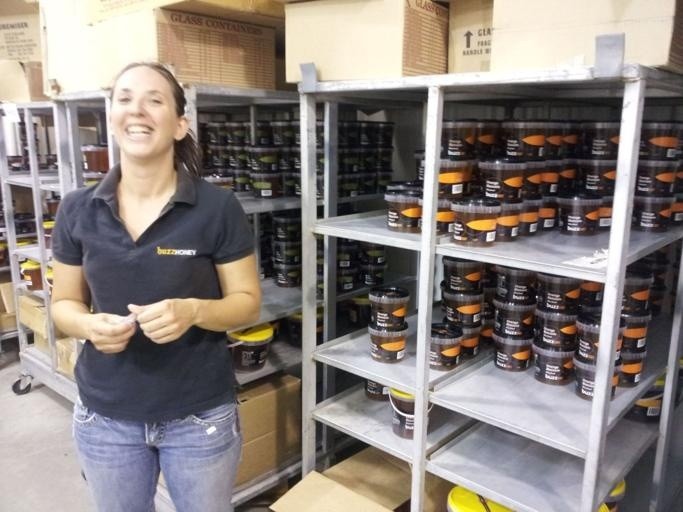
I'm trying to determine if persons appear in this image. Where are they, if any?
[50,64,261,512]
